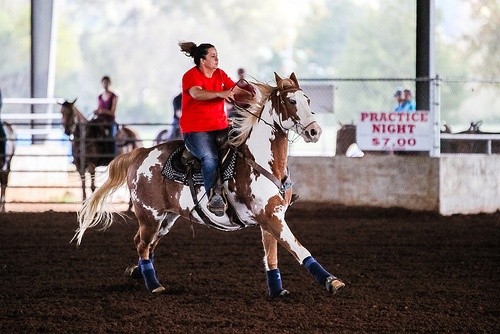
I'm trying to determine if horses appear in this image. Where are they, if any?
[58,98,138,211]
[70,72,345,296]
[336,119,500,156]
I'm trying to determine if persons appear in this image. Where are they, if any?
[178,42,300,217]
[393,90,417,111]
[173,92,182,139]
[93,76,118,165]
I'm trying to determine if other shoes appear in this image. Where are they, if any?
[210,194,224,217]
[290,194,299,203]
